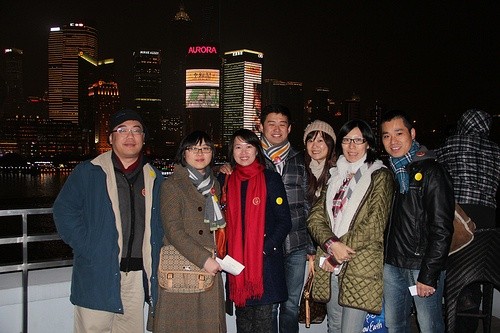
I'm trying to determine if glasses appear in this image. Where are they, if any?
[187,146,212,153]
[341,138,367,145]
[111,127,143,135]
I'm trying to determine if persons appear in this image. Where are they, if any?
[216,106,393,333]
[380,107,455,333]
[431,109,500,312]
[146,130,227,333]
[52,110,164,333]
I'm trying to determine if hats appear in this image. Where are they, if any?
[303,119,336,146]
[108,109,145,136]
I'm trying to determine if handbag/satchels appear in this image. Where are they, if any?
[448,203,477,256]
[299,256,327,328]
[157,245,214,294]
